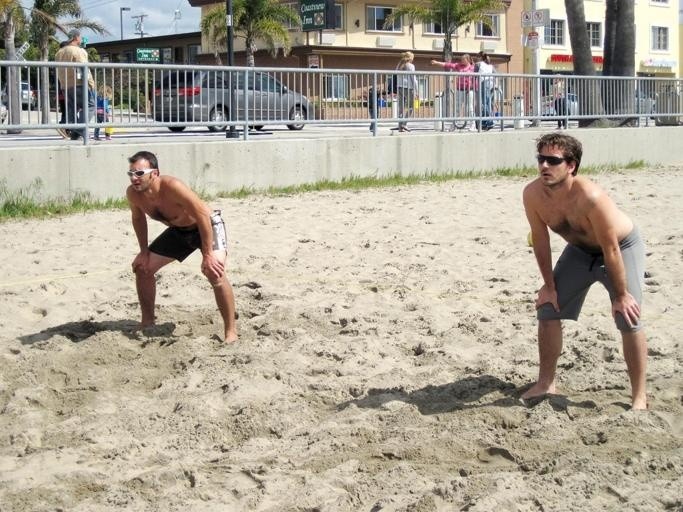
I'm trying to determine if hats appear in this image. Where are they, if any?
[67,28,81,41]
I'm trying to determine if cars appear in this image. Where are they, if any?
[635,92,658,119]
[1,82,37,110]
[541,94,579,116]
[151,68,314,132]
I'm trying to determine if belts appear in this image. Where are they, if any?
[456,88,473,91]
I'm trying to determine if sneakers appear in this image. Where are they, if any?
[56,128,84,140]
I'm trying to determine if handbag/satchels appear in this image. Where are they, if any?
[387,74,397,94]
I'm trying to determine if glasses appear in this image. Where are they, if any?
[538,154,570,165]
[126,168,158,177]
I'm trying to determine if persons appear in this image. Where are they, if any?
[394,51,419,133]
[124,150,240,346]
[430,53,479,132]
[52,40,79,125]
[466,51,498,131]
[552,74,571,130]
[92,84,113,140]
[54,29,95,141]
[520,132,648,413]
